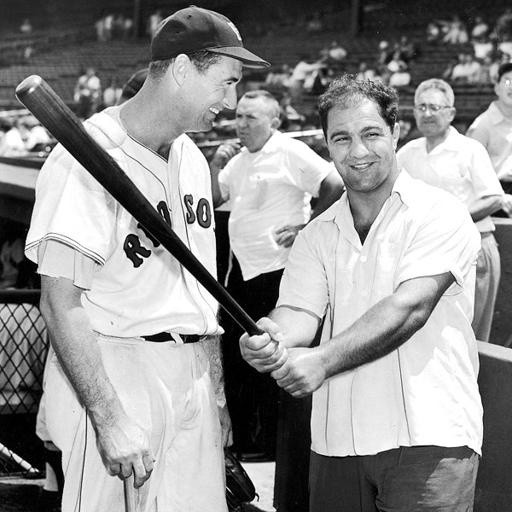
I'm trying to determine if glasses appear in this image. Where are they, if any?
[413,105,453,112]
[500,79,512,87]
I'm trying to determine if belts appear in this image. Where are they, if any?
[139,331,212,344]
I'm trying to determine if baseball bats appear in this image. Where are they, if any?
[16,74,264,336]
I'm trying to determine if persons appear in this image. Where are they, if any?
[25,8,229,512]
[205,61,512,510]
[0,1,511,160]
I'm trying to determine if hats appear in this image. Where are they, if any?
[150,4,272,70]
[497,63,512,83]
[127,69,149,93]
